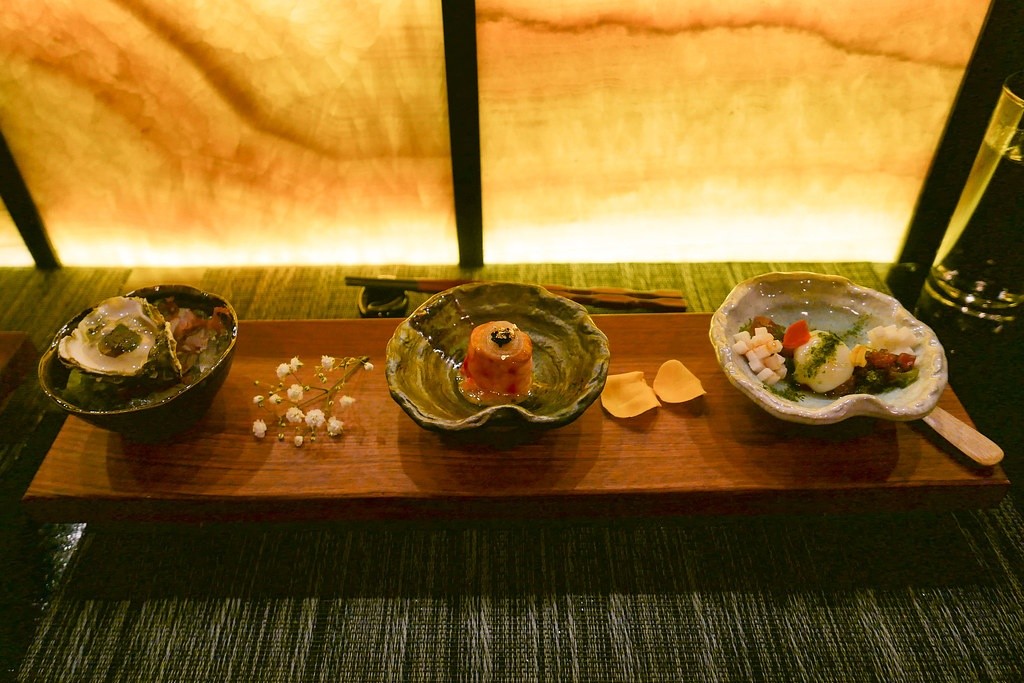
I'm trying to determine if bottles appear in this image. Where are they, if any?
[913,68,1024,393]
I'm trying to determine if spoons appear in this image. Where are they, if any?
[921,403,1005,468]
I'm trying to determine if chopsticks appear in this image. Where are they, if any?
[344,276,690,312]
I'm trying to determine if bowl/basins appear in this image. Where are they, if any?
[34,283,236,442]
[707,266,948,426]
[383,280,611,433]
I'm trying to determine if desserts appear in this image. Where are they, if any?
[455,321,534,406]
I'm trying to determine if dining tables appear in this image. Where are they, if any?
[2,263,1024,683]
